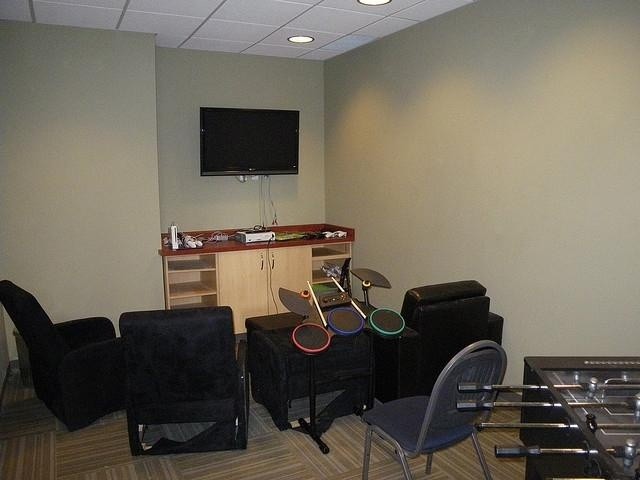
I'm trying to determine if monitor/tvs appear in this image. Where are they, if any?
[200,107,299,176]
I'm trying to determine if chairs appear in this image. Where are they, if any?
[119,306,250,456]
[378,280,504,404]
[0,280,127,432]
[361,339,507,480]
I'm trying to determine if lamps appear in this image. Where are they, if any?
[288,36,314,43]
[357,0,392,6]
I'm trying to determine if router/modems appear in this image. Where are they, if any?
[168,225,179,250]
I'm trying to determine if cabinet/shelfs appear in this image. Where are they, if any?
[158,223,356,335]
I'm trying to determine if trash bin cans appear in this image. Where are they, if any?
[12,327,33,385]
